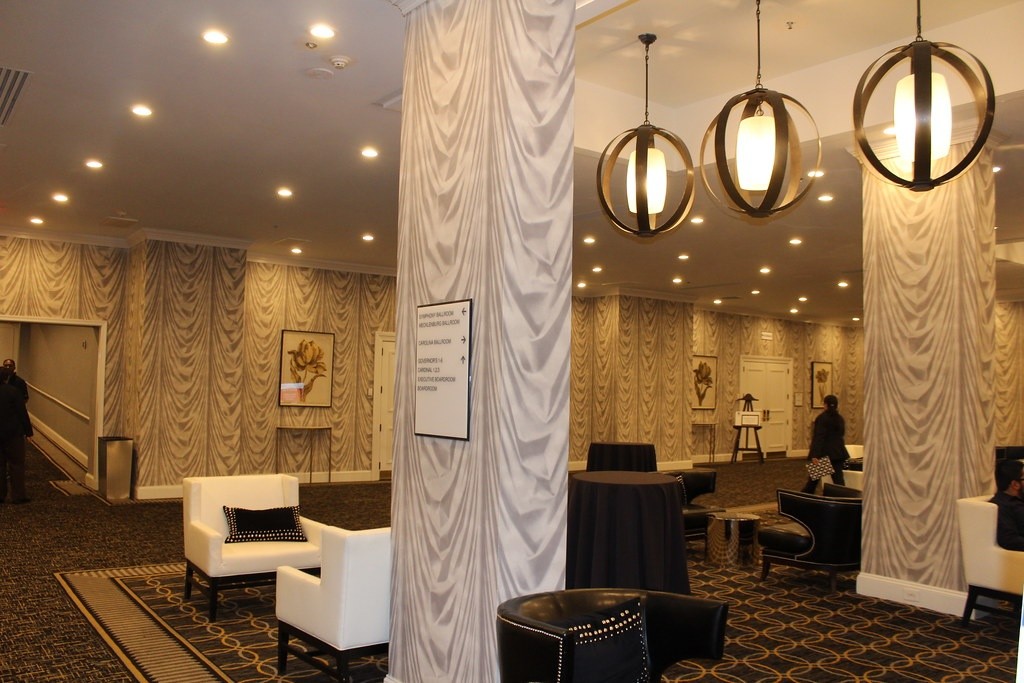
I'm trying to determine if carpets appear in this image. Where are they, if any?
[53,561,389,683]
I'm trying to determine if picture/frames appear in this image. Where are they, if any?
[279,329,335,408]
[811,361,833,409]
[692,354,718,410]
[794,391,803,407]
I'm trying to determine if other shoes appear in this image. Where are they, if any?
[11,496,30,505]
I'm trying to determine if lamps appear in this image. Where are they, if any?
[699,0,822,218]
[852,0,995,192]
[596,34,695,237]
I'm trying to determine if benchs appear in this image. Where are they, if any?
[822,444,864,491]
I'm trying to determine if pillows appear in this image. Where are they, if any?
[550,596,651,683]
[676,476,687,506]
[223,505,308,542]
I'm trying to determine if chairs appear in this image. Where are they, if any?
[183,474,328,623]
[497,588,729,683]
[956,495,1024,628]
[758,483,863,581]
[660,468,718,540]
[276,526,391,683]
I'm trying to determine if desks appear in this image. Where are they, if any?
[731,426,764,464]
[706,511,760,568]
[566,442,691,596]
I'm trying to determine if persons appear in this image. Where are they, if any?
[987,460,1024,551]
[0,359,33,504]
[800,395,850,494]
